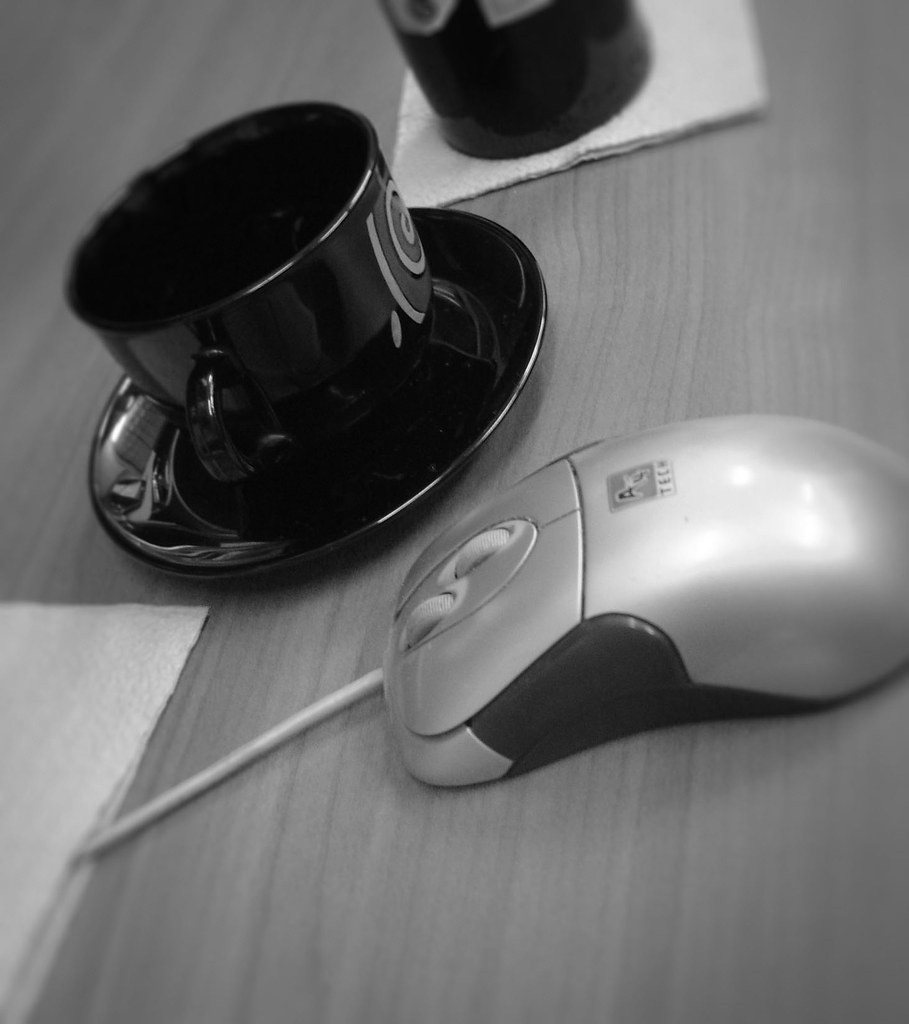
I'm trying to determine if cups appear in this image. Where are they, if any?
[378,0,650,162]
[65,101,432,486]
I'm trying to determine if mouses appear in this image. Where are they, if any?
[378,411,909,789]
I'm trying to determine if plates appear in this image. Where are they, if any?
[87,206,548,580]
